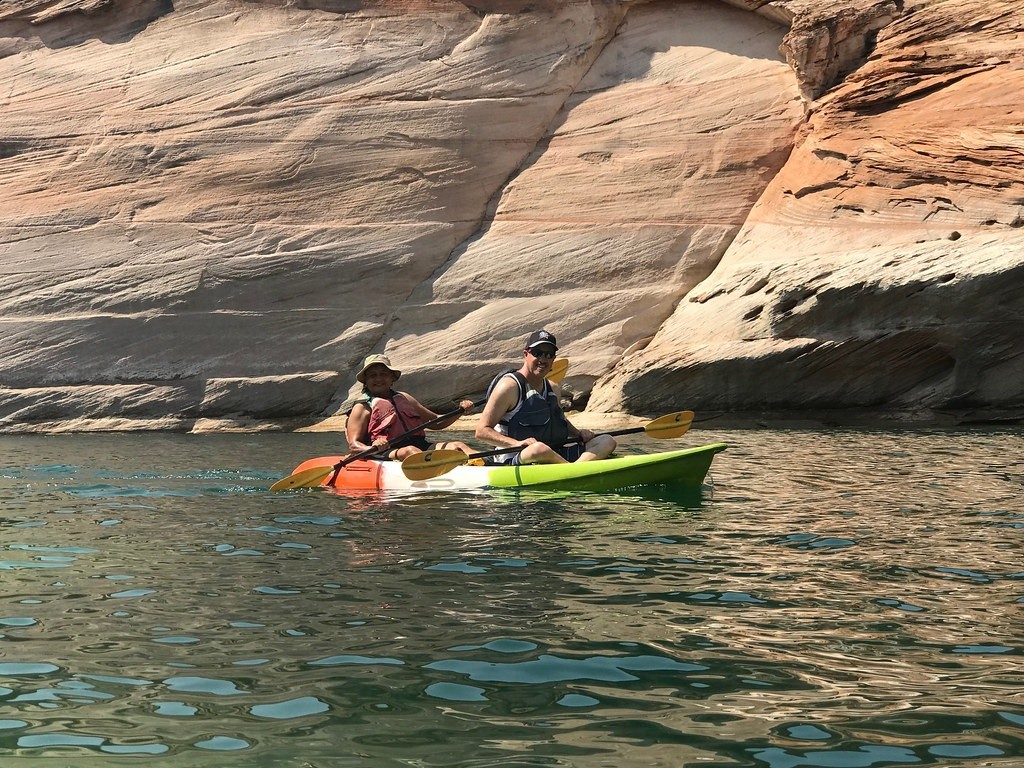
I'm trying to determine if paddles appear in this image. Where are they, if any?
[401,411,696,481]
[269,358,569,490]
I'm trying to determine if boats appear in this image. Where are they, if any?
[290,441,730,498]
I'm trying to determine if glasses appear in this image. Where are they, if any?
[527,348,555,359]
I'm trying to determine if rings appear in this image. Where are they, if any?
[373,442,374,444]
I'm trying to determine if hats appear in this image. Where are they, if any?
[525,330,559,351]
[356,353,401,383]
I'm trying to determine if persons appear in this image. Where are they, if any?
[474,330,617,466]
[347,355,486,468]
